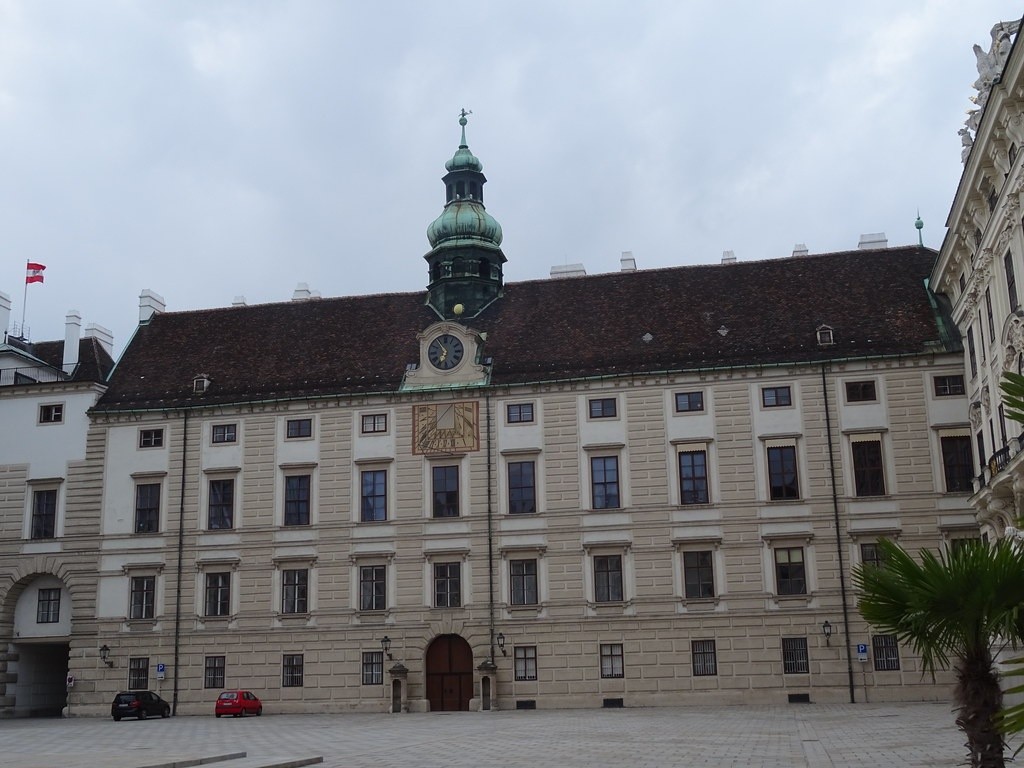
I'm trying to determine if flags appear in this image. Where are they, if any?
[26,262,47,284]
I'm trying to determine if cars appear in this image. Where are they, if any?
[215,690,262,717]
[111,690,170,720]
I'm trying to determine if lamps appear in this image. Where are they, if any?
[381,636,392,661]
[99,645,113,668]
[497,632,506,657]
[823,620,831,647]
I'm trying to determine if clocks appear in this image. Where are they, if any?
[428,334,464,370]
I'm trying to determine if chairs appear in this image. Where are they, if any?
[230,695,234,698]
